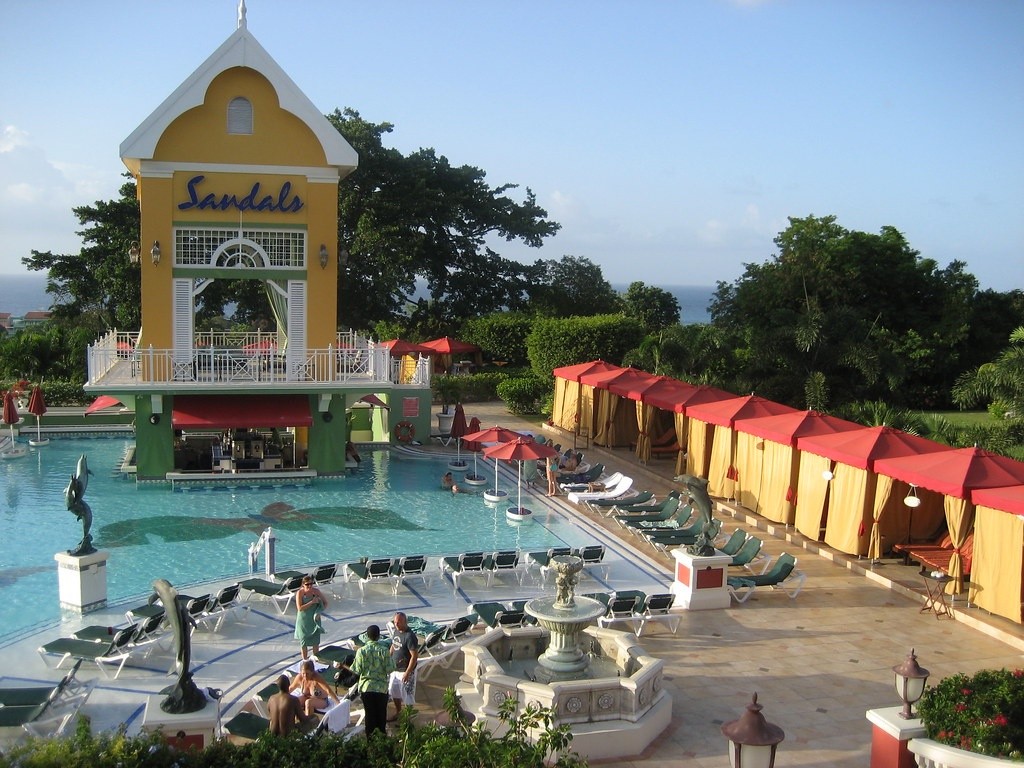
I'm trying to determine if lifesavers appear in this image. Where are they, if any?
[394,421,415,442]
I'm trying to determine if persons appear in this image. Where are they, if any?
[294,576,324,658]
[545,440,576,497]
[269,661,340,736]
[525,434,537,488]
[442,472,460,494]
[334,612,418,742]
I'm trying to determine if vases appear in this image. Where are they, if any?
[15,390,31,409]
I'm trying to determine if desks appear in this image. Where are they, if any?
[918,571,955,621]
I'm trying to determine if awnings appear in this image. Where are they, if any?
[84,396,122,418]
[171,396,314,429]
[361,394,392,413]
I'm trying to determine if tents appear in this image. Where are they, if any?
[867,443,1024,601]
[579,364,646,448]
[686,393,800,503]
[734,407,869,529]
[643,385,739,476]
[117,337,484,376]
[967,484,1024,627]
[794,421,957,560]
[552,358,620,435]
[606,373,693,463]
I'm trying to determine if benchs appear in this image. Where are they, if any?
[196,345,248,371]
[337,349,363,373]
[262,353,286,372]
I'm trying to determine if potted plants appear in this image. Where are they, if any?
[431,375,465,432]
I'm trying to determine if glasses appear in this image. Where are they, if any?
[304,581,314,586]
[395,612,404,615]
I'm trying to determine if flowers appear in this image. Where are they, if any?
[14,380,30,391]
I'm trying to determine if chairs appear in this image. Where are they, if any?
[0,427,806,746]
[211,445,231,470]
[891,523,975,582]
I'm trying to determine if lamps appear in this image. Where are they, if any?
[128,241,140,265]
[892,647,930,720]
[319,244,328,270]
[150,240,161,267]
[720,691,785,768]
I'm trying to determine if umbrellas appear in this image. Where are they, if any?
[28,386,50,445]
[463,417,487,484]
[448,401,469,470]
[461,425,525,501]
[481,437,555,521]
[2,394,25,458]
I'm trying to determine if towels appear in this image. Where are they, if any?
[317,699,351,736]
[293,595,328,648]
